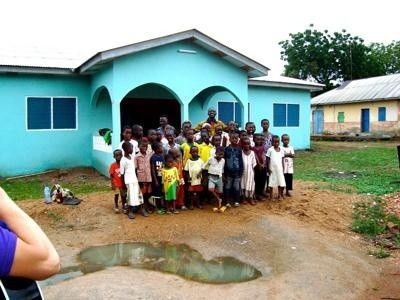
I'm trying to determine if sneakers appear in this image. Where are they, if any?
[181,202,203,211]
[115,206,154,219]
[212,192,292,213]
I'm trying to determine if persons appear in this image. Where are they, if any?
[107,114,295,219]
[0,183,62,299]
[193,106,227,135]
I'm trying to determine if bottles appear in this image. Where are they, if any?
[55,189,62,203]
[43,185,51,203]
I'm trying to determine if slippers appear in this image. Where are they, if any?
[154,207,180,216]
